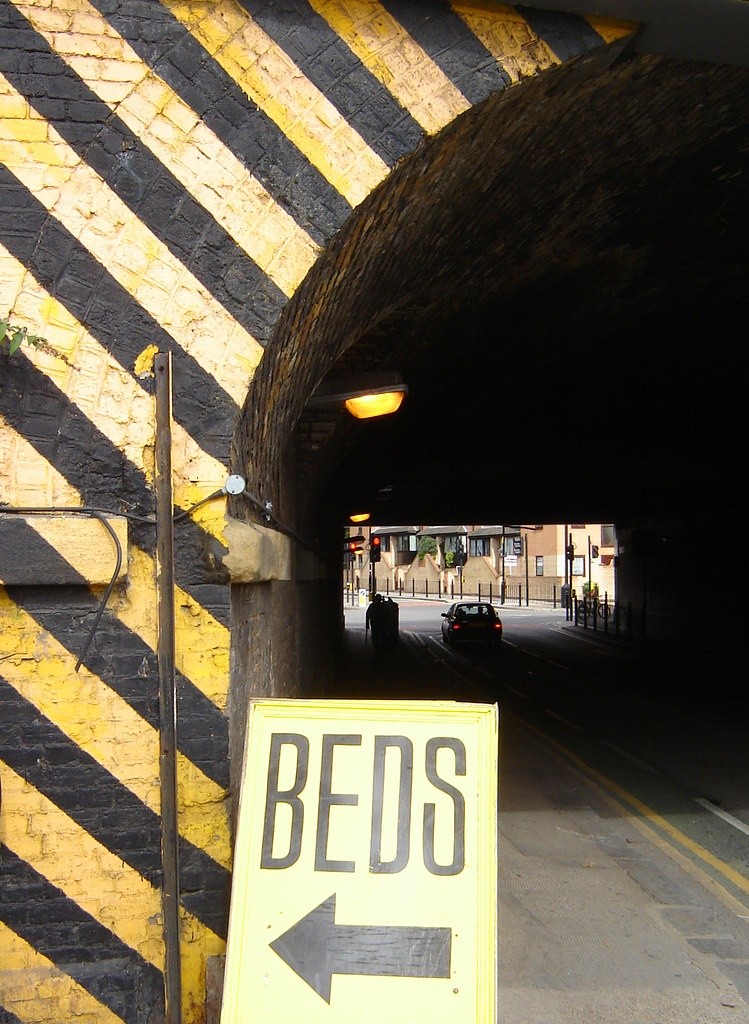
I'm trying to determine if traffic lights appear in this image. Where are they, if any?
[370,536,381,562]
[458,545,464,557]
[566,546,574,561]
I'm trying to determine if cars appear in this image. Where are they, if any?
[441,600,502,652]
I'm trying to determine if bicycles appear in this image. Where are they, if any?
[577,596,611,620]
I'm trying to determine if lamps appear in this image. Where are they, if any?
[304,369,408,418]
